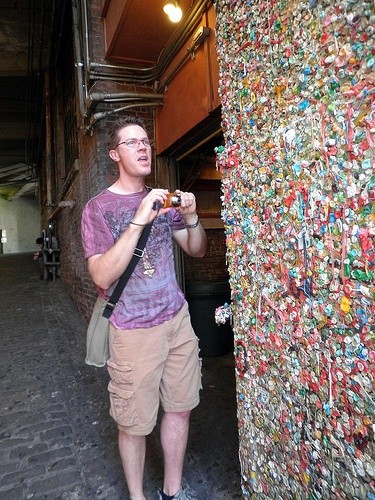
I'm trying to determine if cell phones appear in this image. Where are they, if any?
[153,193,181,210]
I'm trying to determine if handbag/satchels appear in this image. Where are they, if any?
[85,296,109,367]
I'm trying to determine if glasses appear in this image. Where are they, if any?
[113,139,152,148]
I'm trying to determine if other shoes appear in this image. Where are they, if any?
[157,488,200,500]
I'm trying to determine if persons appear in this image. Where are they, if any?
[81,117,207,500]
[32,237,60,281]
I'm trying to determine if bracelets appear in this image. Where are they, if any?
[129,220,146,226]
[185,216,200,228]
